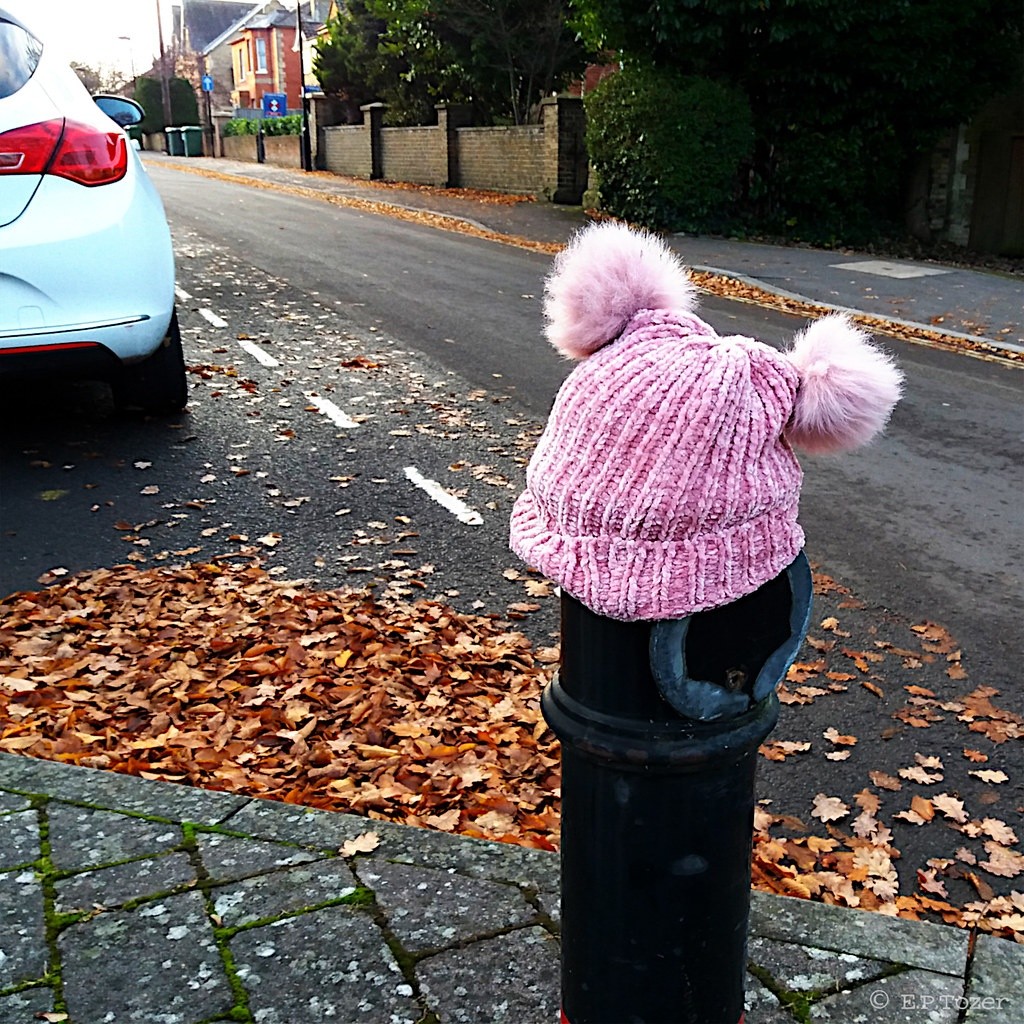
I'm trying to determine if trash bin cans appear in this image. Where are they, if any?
[123,125,145,151]
[165,127,185,156]
[180,126,205,157]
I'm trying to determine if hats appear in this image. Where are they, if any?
[509,220,904,621]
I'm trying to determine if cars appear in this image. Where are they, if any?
[1,8,189,424]
